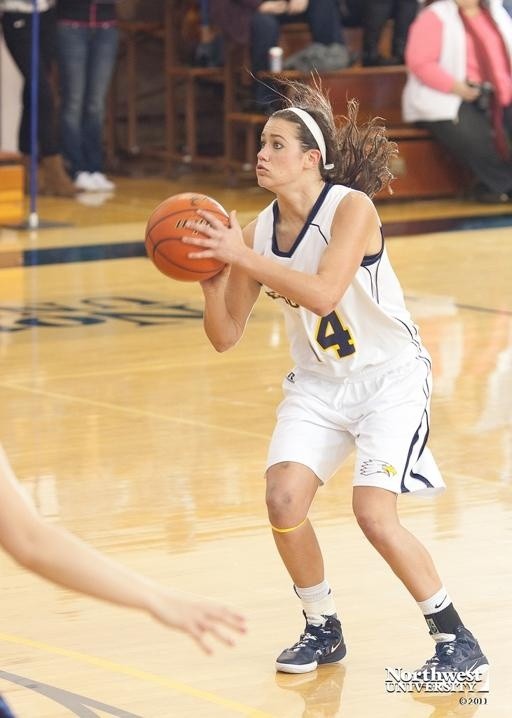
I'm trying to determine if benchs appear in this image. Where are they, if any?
[231,20,458,203]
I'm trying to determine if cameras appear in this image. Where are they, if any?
[468,81,496,113]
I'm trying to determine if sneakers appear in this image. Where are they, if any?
[274,612,346,674]
[73,171,115,192]
[409,625,490,683]
[360,42,407,67]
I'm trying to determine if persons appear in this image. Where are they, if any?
[1,0,89,202]
[177,77,494,690]
[0,454,250,655]
[55,0,117,193]
[401,1,512,203]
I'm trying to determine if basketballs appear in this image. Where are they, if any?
[146,192,231,282]
